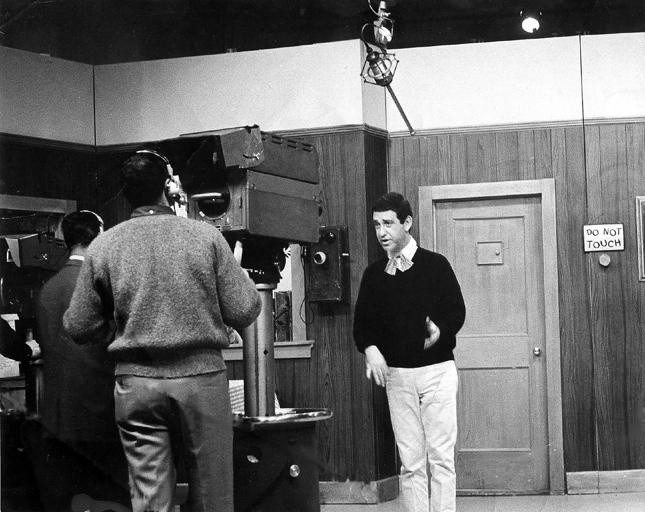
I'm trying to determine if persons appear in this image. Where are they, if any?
[352,191,466,512]
[29,210,129,511]
[58,151,265,511]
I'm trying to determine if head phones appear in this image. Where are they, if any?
[52,206,107,245]
[135,146,182,206]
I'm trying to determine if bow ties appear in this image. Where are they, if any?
[384,253,414,277]
